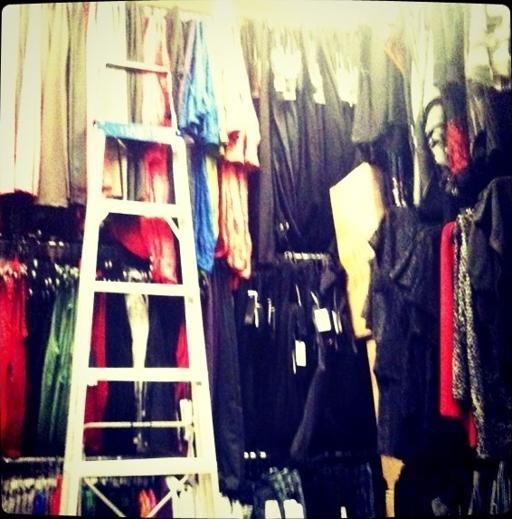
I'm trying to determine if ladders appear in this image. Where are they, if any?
[60,56,221,519]
